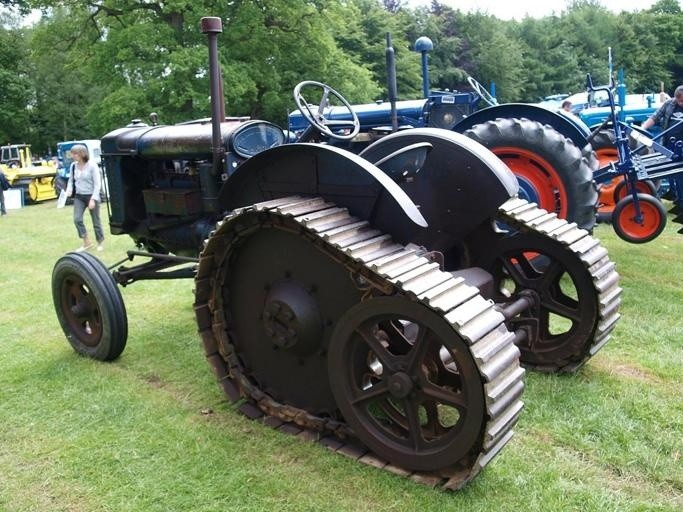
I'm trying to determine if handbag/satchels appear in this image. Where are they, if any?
[70,180,76,198]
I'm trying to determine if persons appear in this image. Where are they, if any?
[641,85,683,144]
[64,144,105,253]
[560,101,572,112]
[0,172,10,215]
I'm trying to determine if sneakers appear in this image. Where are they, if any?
[77,244,104,251]
